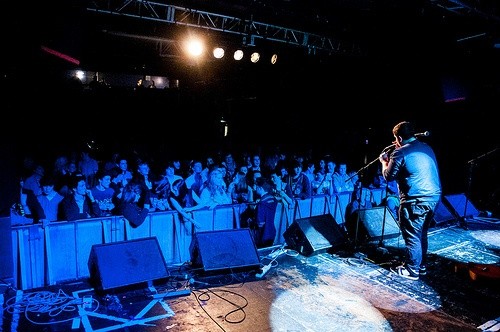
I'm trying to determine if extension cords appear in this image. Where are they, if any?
[255,265,271,278]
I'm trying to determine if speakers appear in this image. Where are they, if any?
[87,236,171,298]
[189,227,261,278]
[431,193,479,227]
[283,213,349,257]
[345,207,403,244]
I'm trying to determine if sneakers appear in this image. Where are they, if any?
[401,261,428,276]
[389,265,420,281]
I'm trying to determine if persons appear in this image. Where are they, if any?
[380,121,440,280]
[247,178,279,249]
[10,150,400,229]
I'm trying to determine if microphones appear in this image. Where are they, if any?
[385,144,396,150]
[414,131,430,137]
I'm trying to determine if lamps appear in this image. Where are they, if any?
[211,45,225,59]
[260,51,279,65]
[243,50,261,64]
[228,47,244,61]
